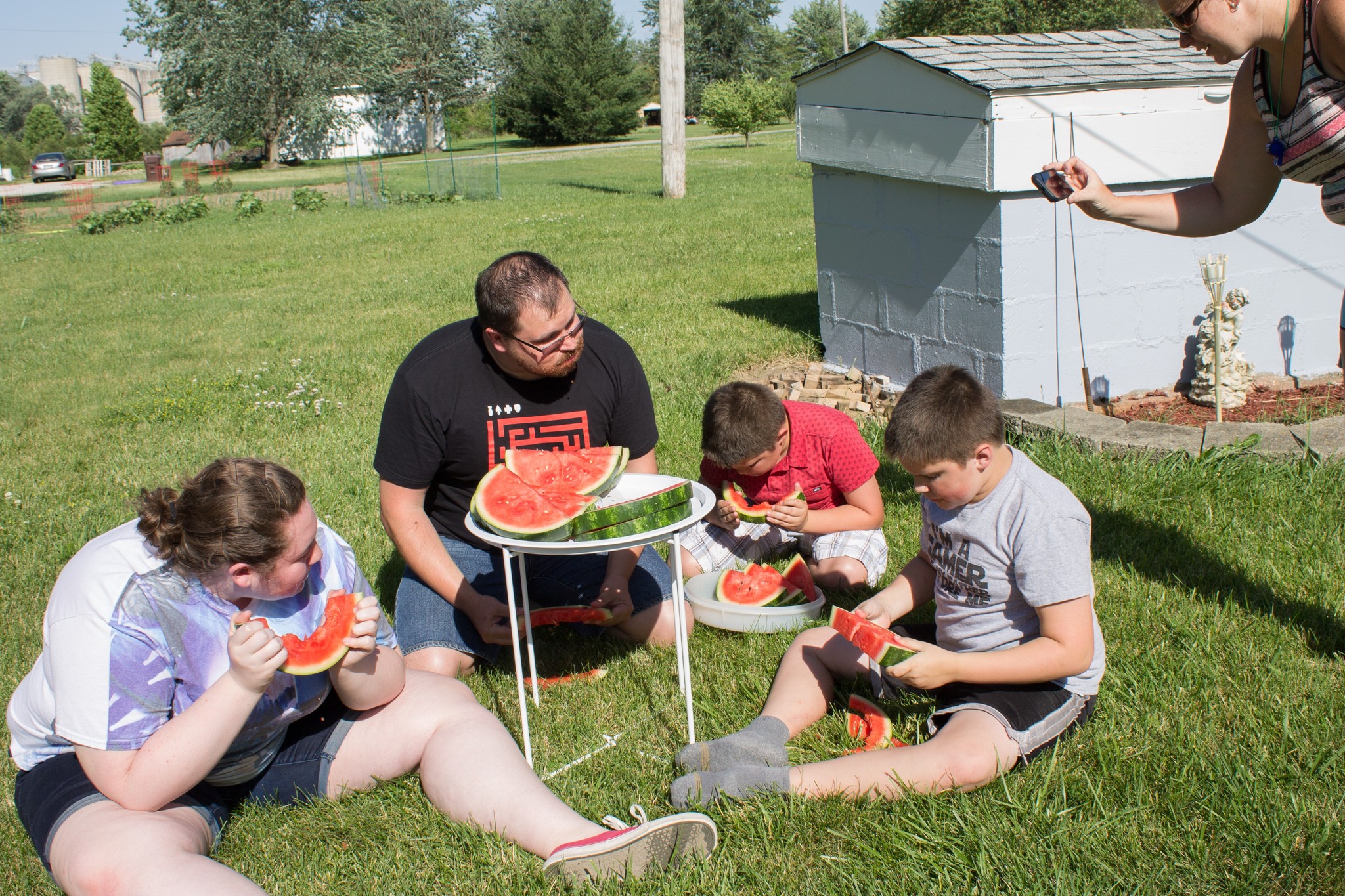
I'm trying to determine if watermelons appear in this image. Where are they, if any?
[504,445,630,497]
[572,481,692,540]
[235,592,363,676]
[782,552,817,603]
[470,464,603,542]
[829,605,918,667]
[716,561,811,606]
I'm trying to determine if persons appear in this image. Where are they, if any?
[373,249,695,686]
[1042,0,1345,661]
[667,366,1109,806]
[670,384,887,591]
[5,460,722,896]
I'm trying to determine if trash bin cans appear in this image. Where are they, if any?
[143,155,162,181]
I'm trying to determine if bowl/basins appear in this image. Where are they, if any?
[683,570,827,635]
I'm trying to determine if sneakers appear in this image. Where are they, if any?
[542,805,717,886]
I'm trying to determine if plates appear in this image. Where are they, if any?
[464,473,716,556]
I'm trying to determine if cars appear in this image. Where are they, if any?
[30,152,75,184]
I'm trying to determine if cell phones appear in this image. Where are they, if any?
[1032,168,1076,203]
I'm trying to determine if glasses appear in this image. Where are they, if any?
[505,300,588,359]
[1167,0,1202,35]
